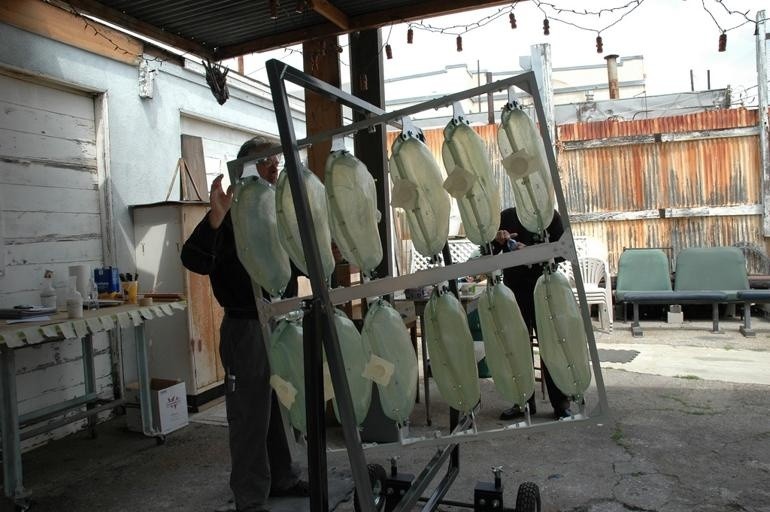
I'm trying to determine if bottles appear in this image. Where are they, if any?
[86,278,99,315]
[39,270,57,313]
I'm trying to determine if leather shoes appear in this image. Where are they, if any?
[554,405,573,421]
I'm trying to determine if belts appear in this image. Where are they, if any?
[224,308,261,320]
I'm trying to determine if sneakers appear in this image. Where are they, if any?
[499,400,537,422]
[268,477,311,500]
[237,502,277,512]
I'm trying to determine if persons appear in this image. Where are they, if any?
[181,135,309,512]
[479,209,575,422]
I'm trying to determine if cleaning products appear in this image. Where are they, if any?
[40,269,58,308]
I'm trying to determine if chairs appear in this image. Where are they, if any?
[568,246,770,341]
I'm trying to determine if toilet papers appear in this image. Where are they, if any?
[68,263,93,299]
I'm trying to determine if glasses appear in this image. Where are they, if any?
[255,156,285,172]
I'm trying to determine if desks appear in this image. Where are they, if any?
[0,297,182,498]
[403,280,490,425]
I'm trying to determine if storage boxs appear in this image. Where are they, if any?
[122,376,190,435]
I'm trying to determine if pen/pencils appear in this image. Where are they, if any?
[119,272,139,281]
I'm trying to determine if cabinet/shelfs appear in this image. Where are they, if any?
[126,192,231,422]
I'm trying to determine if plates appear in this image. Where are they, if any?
[69,298,125,307]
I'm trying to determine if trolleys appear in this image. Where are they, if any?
[263,57,542,511]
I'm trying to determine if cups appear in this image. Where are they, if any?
[119,279,139,305]
